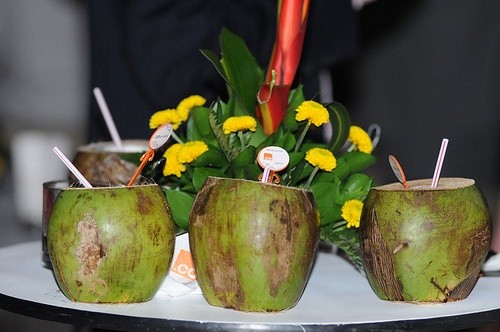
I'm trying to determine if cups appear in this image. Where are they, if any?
[41,179,115,270]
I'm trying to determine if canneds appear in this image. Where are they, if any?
[41,180,71,269]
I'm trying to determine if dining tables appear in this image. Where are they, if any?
[1,239,500,331]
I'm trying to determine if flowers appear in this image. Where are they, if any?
[144,28,378,272]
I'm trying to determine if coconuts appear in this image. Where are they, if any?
[48,184,177,303]
[360,177,492,304]
[69,139,152,186]
[188,176,320,314]
